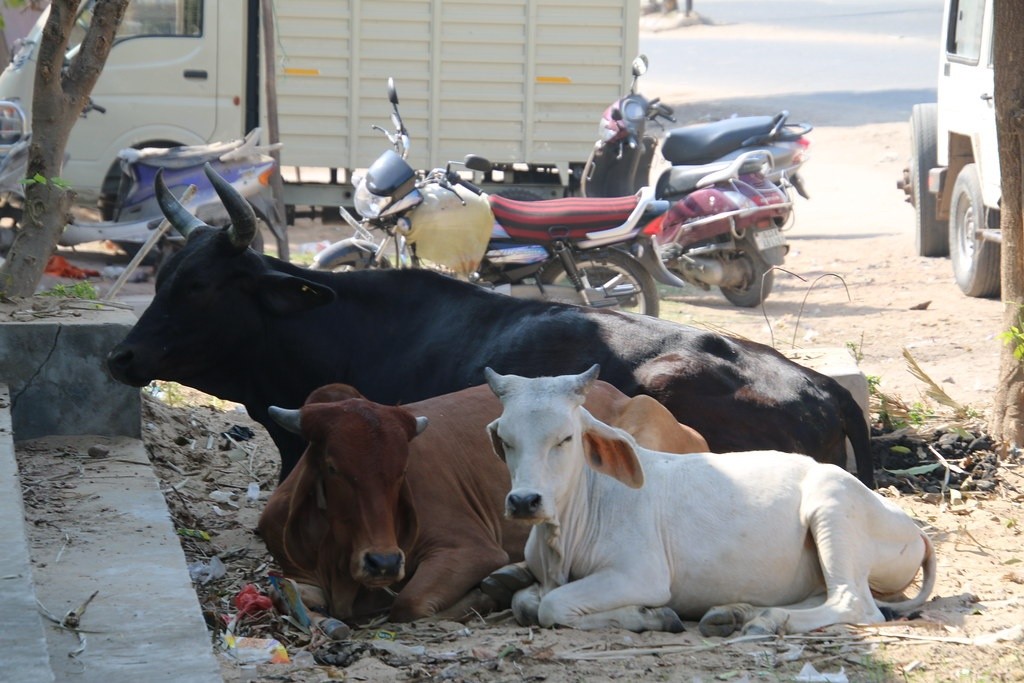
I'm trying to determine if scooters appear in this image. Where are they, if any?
[581,55,815,309]
[0,93,286,272]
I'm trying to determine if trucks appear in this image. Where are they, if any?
[895,0,1004,299]
[1,0,640,269]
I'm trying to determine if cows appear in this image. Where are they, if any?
[105,159,937,635]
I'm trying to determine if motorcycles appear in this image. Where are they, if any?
[308,77,687,319]
